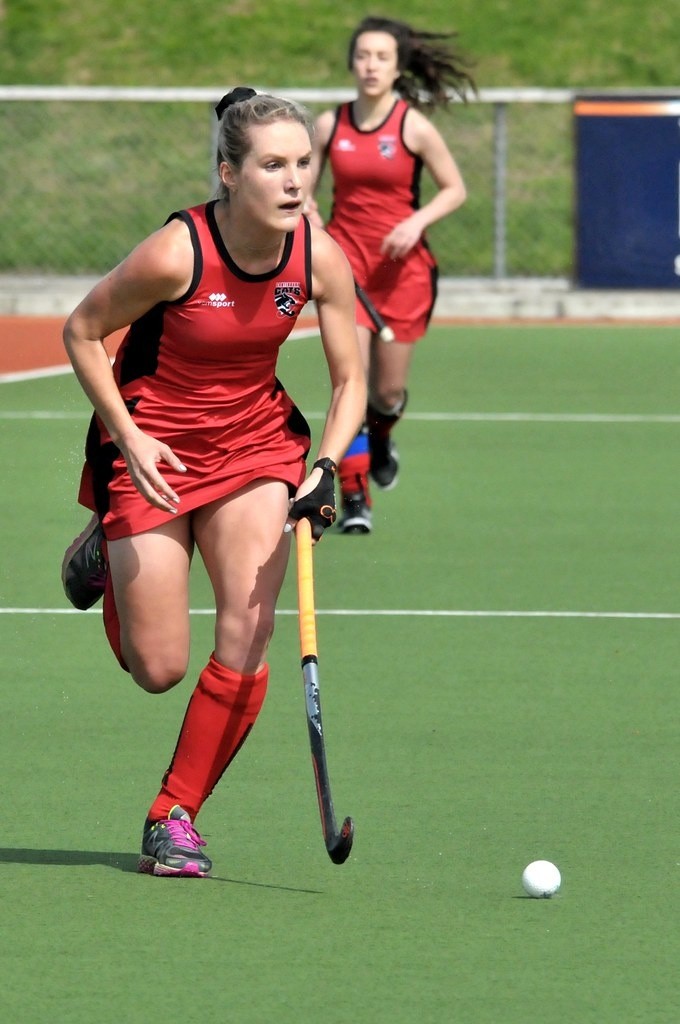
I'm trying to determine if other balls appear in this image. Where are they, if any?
[521,859,562,898]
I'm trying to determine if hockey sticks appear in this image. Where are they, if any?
[295,515,355,865]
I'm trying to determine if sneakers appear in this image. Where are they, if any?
[367,432,402,490]
[61,511,110,611]
[137,805,213,878]
[337,492,373,533]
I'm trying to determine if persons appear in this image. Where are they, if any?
[61,87,367,877]
[309,16,478,533]
